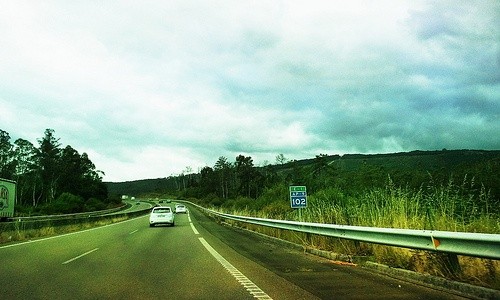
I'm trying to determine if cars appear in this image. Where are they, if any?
[149,206,176,228]
[121,194,187,214]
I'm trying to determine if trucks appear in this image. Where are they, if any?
[0,178,17,219]
